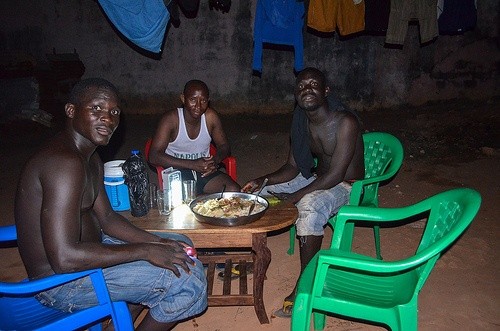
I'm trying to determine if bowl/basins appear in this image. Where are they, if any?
[189,191,270,227]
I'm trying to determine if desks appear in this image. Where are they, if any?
[113,193,300,325]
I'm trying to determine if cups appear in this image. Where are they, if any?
[156,188,171,216]
[182,180,196,206]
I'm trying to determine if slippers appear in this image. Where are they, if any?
[218,264,253,281]
[271,301,293,318]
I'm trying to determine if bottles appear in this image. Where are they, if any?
[121,150,151,217]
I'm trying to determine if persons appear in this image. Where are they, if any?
[15,78,209,331]
[148,79,241,196]
[218,67,365,318]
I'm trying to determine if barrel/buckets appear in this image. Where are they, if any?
[103,159,131,212]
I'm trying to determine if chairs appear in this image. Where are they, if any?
[290,188,482,331]
[142,130,238,192]
[0,223,134,331]
[286,132,404,261]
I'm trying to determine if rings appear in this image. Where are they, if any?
[204,166,208,170]
